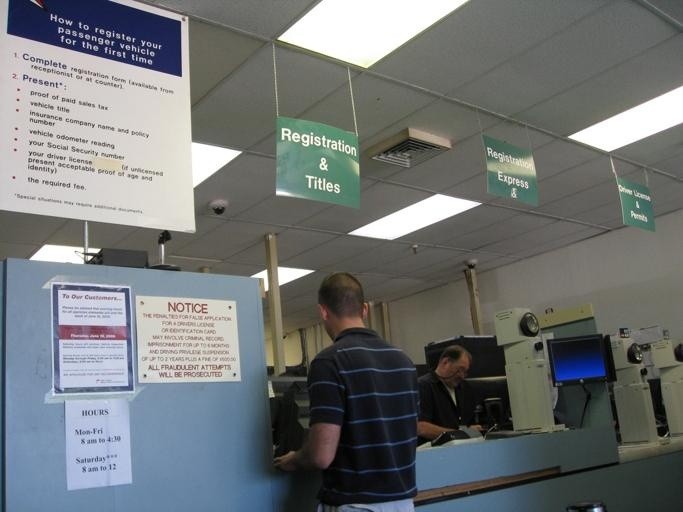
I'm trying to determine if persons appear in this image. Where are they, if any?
[276,270,419,512]
[414,346,483,441]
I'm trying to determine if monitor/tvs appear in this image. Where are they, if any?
[546,334,614,387]
[604,336,618,382]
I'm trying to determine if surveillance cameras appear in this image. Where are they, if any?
[466,258,477,268]
[208,200,228,216]
